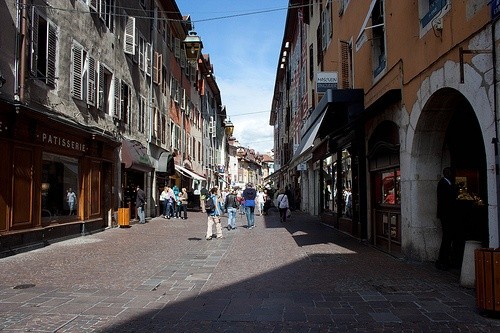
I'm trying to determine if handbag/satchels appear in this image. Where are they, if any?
[160,195,165,201]
[182,200,188,206]
[278,202,280,208]
[286,209,291,218]
[233,197,237,208]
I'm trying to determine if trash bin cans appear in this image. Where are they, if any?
[118,207,130,226]
[474,248,500,318]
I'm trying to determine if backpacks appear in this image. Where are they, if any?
[205,196,215,212]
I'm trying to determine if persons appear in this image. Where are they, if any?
[67,188,77,216]
[200,184,298,223]
[159,187,188,220]
[224,189,239,230]
[205,188,223,240]
[136,186,146,224]
[241,182,257,229]
[277,188,289,224]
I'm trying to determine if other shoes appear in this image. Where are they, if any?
[162,214,188,220]
[228,224,231,230]
[252,225,255,228]
[247,226,252,229]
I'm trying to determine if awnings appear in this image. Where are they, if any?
[288,106,327,166]
[175,165,206,181]
